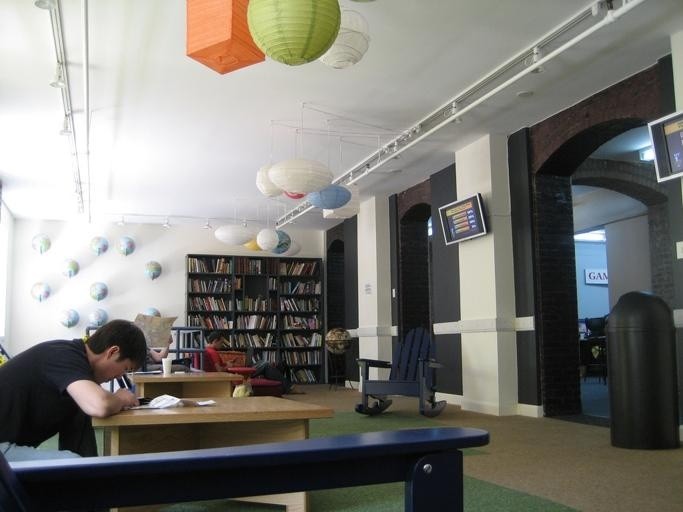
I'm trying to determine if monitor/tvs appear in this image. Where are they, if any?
[646,112,679,183]
[436,193,489,247]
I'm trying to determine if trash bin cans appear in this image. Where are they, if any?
[604,291,680,451]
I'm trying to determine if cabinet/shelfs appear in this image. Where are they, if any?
[325,340,355,392]
[185,254,323,384]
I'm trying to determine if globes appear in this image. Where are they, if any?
[325,328,356,392]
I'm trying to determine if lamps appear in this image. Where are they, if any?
[250,105,356,212]
[180,0,379,77]
[214,199,294,254]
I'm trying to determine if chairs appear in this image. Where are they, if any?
[583,317,604,383]
[579,339,605,384]
[0,425,489,512]
[195,346,281,396]
[355,328,446,417]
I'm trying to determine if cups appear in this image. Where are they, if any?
[161,358,173,377]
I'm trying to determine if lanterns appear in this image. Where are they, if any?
[256,158,351,209]
[214,224,291,253]
[185,0,368,75]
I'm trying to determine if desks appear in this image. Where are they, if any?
[89,396,335,512]
[124,372,242,398]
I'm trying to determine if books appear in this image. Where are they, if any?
[188,257,323,382]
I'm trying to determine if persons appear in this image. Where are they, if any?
[0,319,147,462]
[205,331,305,395]
[145,335,173,362]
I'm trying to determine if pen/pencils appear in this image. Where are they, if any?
[131,384,136,394]
